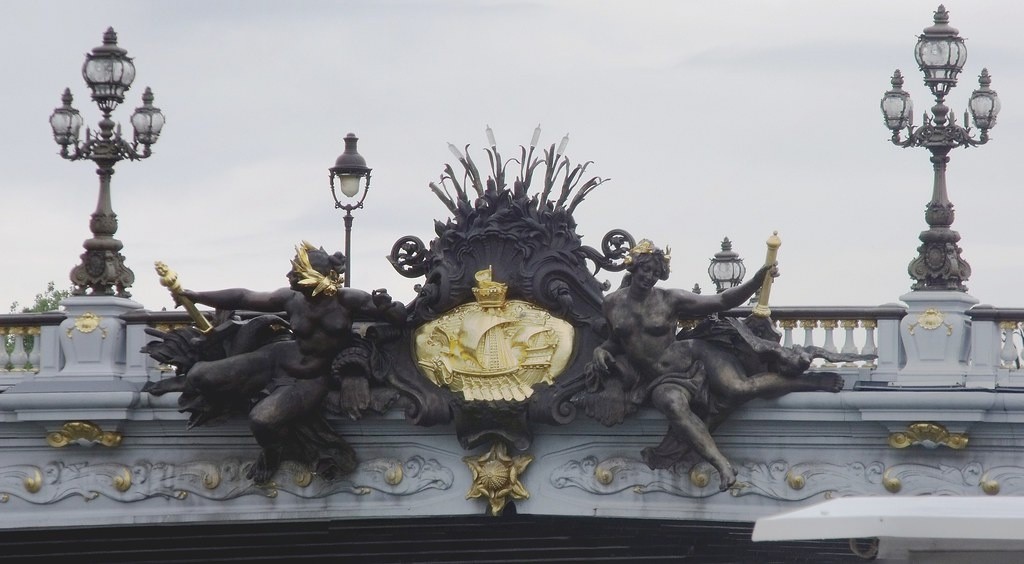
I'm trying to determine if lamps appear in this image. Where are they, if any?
[329,132,372,287]
[49,26,168,300]
[880,2,1002,291]
[709,237,747,292]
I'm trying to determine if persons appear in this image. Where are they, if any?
[174,240,407,483]
[591,239,845,492]
